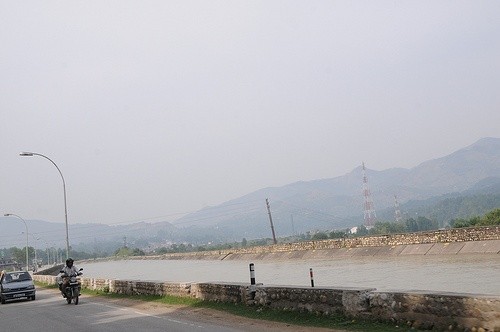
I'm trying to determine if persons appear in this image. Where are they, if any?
[59,258,81,298]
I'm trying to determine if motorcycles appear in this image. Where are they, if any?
[58,268,84,305]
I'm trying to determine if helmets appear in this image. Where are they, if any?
[66,258,74,268]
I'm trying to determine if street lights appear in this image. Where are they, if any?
[3,213,29,271]
[19,151,69,258]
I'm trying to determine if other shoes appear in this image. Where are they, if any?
[79,293,81,296]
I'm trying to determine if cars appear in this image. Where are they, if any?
[0,271,36,304]
